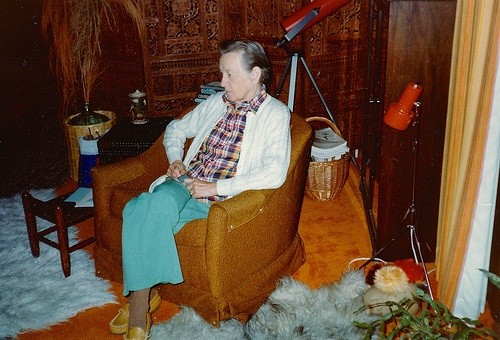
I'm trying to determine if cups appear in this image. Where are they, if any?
[129,90,149,124]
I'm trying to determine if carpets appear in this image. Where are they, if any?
[0,189,120,340]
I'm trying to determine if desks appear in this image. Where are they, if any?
[21,188,97,277]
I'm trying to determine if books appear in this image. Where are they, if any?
[195,81,265,105]
[310,128,350,162]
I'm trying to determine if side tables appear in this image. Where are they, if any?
[98,116,174,165]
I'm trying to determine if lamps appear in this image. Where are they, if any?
[358,81,437,304]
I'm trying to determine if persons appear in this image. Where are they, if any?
[108,39,291,339]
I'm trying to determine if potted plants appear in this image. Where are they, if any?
[39,0,149,184]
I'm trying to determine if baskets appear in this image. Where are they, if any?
[303,117,350,203]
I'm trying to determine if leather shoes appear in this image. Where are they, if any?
[109,290,161,340]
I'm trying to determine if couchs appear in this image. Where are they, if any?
[89,103,316,329]
